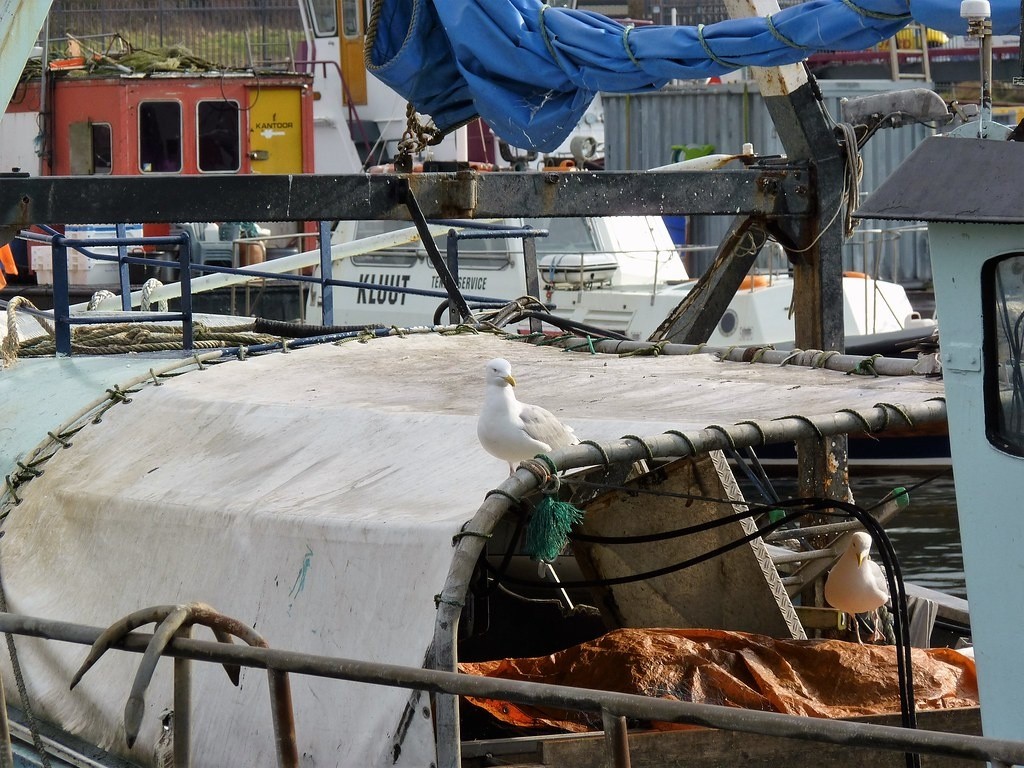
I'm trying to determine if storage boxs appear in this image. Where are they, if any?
[30,246,143,285]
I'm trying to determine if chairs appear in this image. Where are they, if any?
[170,222,233,277]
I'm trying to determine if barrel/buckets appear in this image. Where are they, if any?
[127,251,165,283]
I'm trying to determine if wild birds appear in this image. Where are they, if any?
[476,358,582,476]
[824,532,889,655]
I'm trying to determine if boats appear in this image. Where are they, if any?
[0,137,1024,768]
